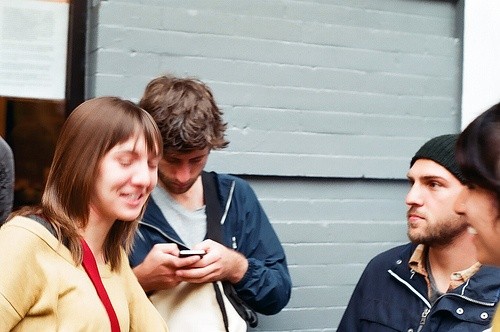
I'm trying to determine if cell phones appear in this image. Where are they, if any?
[179,250,205,258]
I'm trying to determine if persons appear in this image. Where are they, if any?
[335,103,500,332]
[0,96,170,332]
[122,75,292,332]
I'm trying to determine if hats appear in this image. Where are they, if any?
[414,133,469,187]
